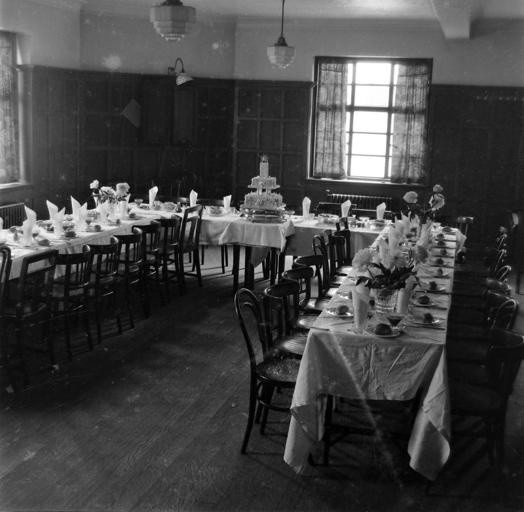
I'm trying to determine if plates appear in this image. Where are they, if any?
[9,197,229,255]
[324,254,381,319]
[361,324,402,337]
[408,227,458,325]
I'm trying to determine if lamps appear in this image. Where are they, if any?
[167,57,195,86]
[150,1,198,41]
[267,1,296,68]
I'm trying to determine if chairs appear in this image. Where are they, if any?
[3,179,523,493]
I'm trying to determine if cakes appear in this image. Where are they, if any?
[244,176,283,210]
